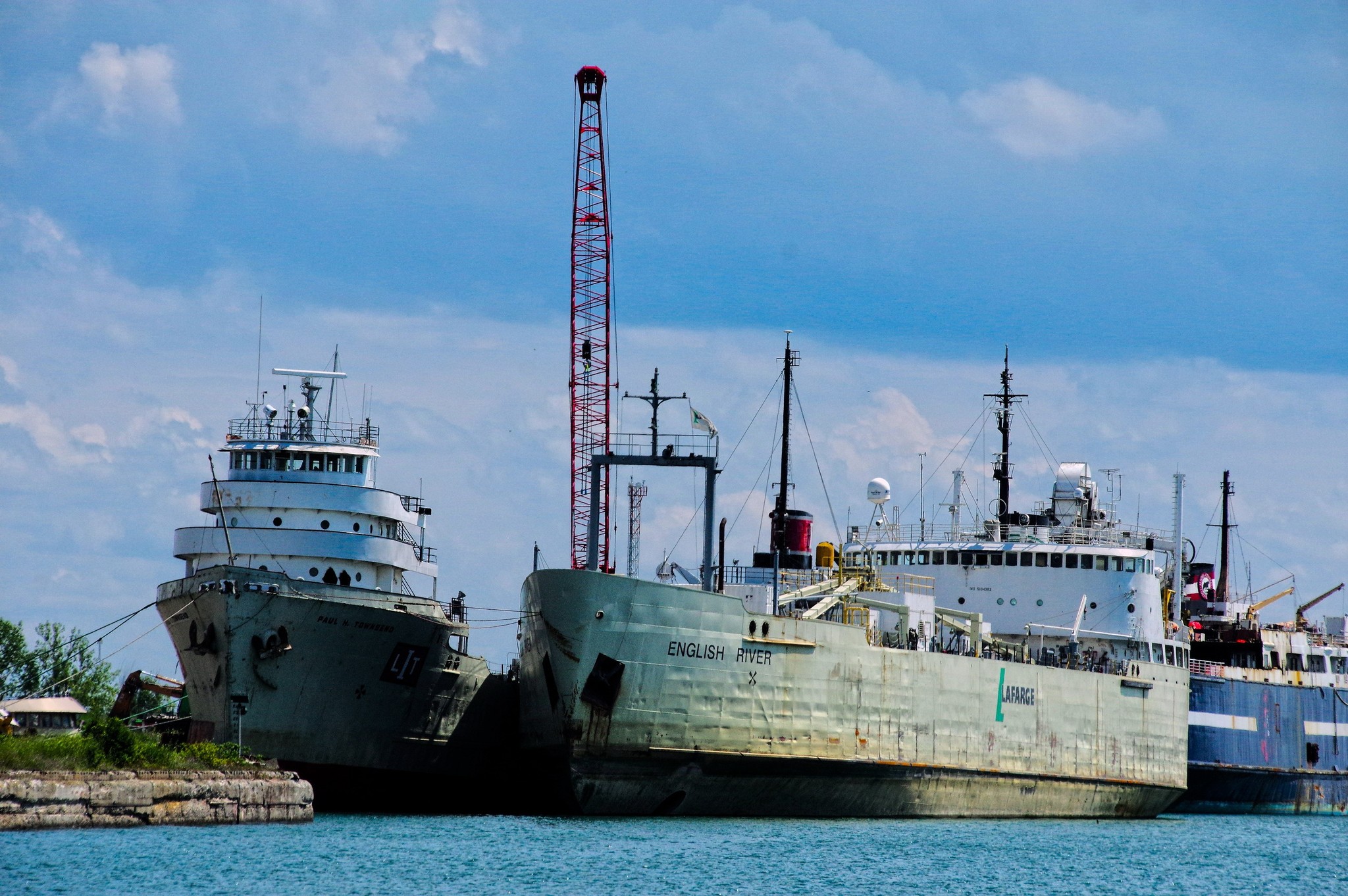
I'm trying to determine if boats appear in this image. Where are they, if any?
[126,292,497,815]
[1169,469,1348,804]
[490,326,1198,820]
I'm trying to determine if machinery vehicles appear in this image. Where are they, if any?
[107,671,191,745]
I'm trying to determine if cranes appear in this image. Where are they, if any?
[568,60,632,573]
